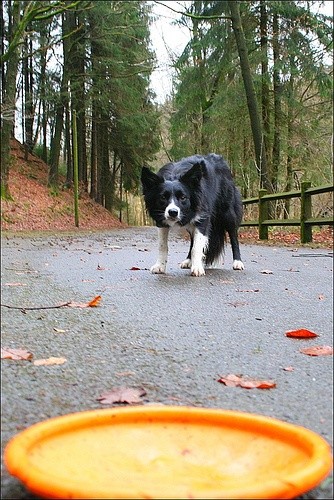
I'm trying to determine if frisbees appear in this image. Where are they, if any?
[2,406,332,500]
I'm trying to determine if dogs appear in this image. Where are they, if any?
[139,150,246,279]
